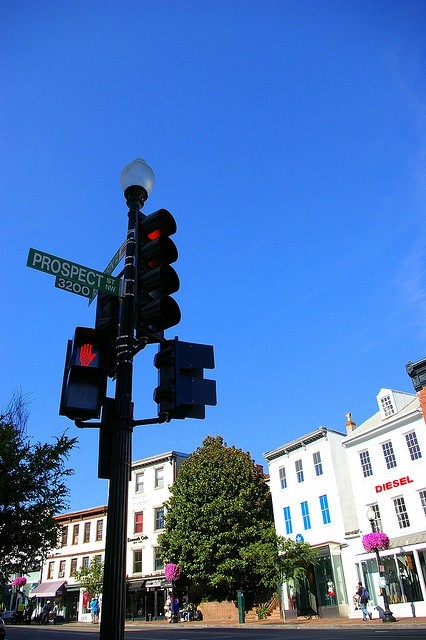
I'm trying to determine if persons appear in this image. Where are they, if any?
[41,600,50,624]
[326,578,335,607]
[387,568,398,603]
[163,591,179,622]
[357,582,372,621]
[354,586,361,610]
[399,568,407,603]
[181,600,202,622]
[288,584,294,609]
[88,596,101,623]
[52,601,58,616]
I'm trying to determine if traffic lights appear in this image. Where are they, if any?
[133,209,181,342]
[153,337,216,419]
[60,327,107,420]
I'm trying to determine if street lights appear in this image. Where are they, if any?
[99,159,155,640]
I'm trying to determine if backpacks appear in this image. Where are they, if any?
[360,587,370,602]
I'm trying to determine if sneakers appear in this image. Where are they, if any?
[363,618,366,621]
[368,612,372,620]
